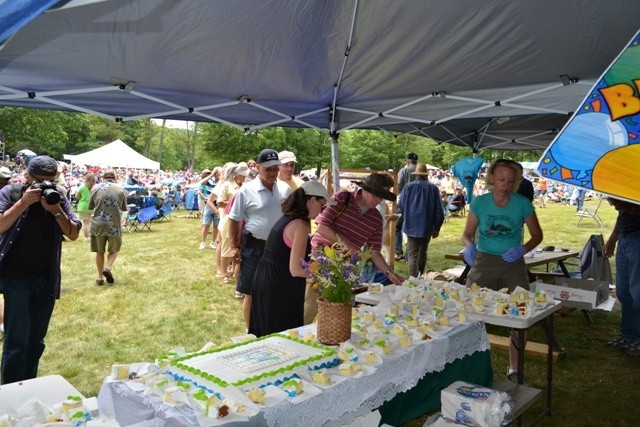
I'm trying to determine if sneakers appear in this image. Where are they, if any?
[84,237,91,242]
[624,339,638,351]
[608,336,630,347]
[96,279,104,285]
[210,242,216,248]
[221,275,229,284]
[505,368,527,386]
[103,268,114,283]
[200,242,206,249]
[395,251,403,260]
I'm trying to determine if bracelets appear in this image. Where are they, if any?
[205,197,208,203]
[386,266,392,276]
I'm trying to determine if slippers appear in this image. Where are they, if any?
[235,290,244,298]
[217,271,221,277]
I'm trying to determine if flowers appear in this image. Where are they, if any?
[298,234,371,302]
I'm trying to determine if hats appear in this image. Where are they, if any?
[351,173,396,201]
[100,170,116,178]
[278,151,298,164]
[301,181,336,206]
[410,164,429,175]
[407,153,418,160]
[28,155,57,175]
[258,149,282,168]
[16,149,36,164]
[235,163,250,175]
[0,166,11,178]
[456,186,463,189]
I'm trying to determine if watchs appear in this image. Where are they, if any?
[53,208,66,218]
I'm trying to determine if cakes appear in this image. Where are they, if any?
[534,287,546,304]
[44,395,90,426]
[152,377,168,389]
[172,333,337,397]
[338,361,359,375]
[516,301,528,320]
[351,306,449,352]
[248,387,266,402]
[496,300,510,318]
[205,395,229,419]
[173,380,190,391]
[363,352,376,362]
[458,311,466,322]
[190,389,207,403]
[159,353,179,365]
[303,332,314,342]
[235,404,247,414]
[311,367,329,383]
[288,330,298,338]
[114,363,130,379]
[390,277,479,307]
[282,378,303,394]
[473,293,486,307]
[510,288,529,302]
[338,346,355,358]
[370,283,383,294]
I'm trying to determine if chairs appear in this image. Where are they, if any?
[574,194,604,228]
[157,203,173,221]
[449,203,466,217]
[129,205,157,232]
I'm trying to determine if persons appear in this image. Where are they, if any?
[462,160,543,385]
[602,199,636,355]
[56,166,69,200]
[0,167,12,334]
[398,164,445,279]
[75,172,96,243]
[448,183,466,219]
[277,150,304,210]
[304,170,405,325]
[533,177,547,208]
[228,150,292,334]
[219,162,239,284]
[198,166,223,251]
[200,168,212,236]
[90,171,128,284]
[517,164,535,202]
[0,155,82,386]
[246,179,338,337]
[396,153,419,262]
[208,161,250,278]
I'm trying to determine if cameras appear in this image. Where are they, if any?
[20,179,61,206]
[15,157,21,165]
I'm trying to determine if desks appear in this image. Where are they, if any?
[445,246,593,350]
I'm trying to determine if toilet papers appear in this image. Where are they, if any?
[440,380,514,427]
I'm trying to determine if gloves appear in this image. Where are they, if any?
[502,247,525,263]
[464,244,476,267]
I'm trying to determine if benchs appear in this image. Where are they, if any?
[424,379,544,427]
